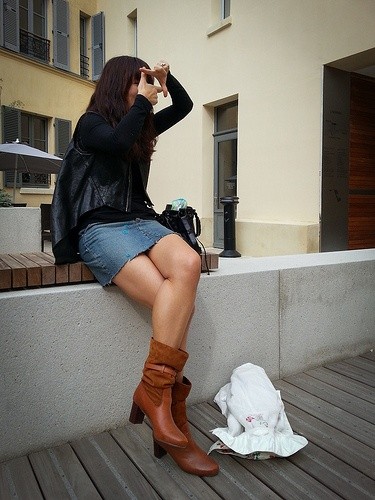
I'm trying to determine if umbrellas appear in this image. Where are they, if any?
[0,138,64,204]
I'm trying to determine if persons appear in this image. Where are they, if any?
[50,56,219,477]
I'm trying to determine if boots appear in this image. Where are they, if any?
[152,375,220,477]
[129,337,189,449]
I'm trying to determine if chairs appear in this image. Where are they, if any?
[40,203,53,252]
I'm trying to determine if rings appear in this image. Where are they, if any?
[161,63,166,66]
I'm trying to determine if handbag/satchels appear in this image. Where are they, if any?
[159,199,209,275]
